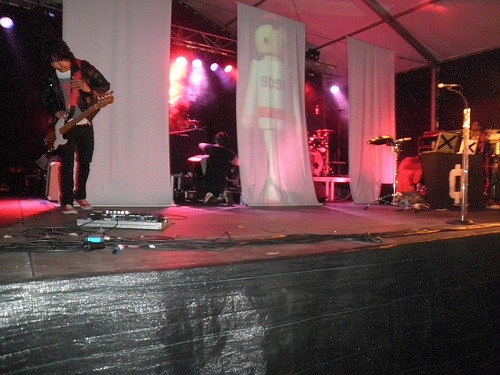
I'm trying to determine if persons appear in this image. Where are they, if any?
[199,132,240,208]
[470,120,487,155]
[40,38,111,214]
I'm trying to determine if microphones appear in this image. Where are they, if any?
[437,83,461,88]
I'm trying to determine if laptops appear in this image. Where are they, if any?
[423,132,458,153]
[456,139,478,154]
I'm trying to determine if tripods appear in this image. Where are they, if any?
[364,146,403,210]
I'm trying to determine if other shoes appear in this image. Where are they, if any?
[415,203,430,210]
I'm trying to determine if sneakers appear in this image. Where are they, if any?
[203,192,213,204]
[224,191,233,206]
[60,204,77,214]
[73,199,91,209]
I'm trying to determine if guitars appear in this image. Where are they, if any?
[44,94,115,153]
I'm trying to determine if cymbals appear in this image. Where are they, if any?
[317,128,336,134]
[368,135,412,145]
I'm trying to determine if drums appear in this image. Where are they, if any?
[308,136,326,175]
